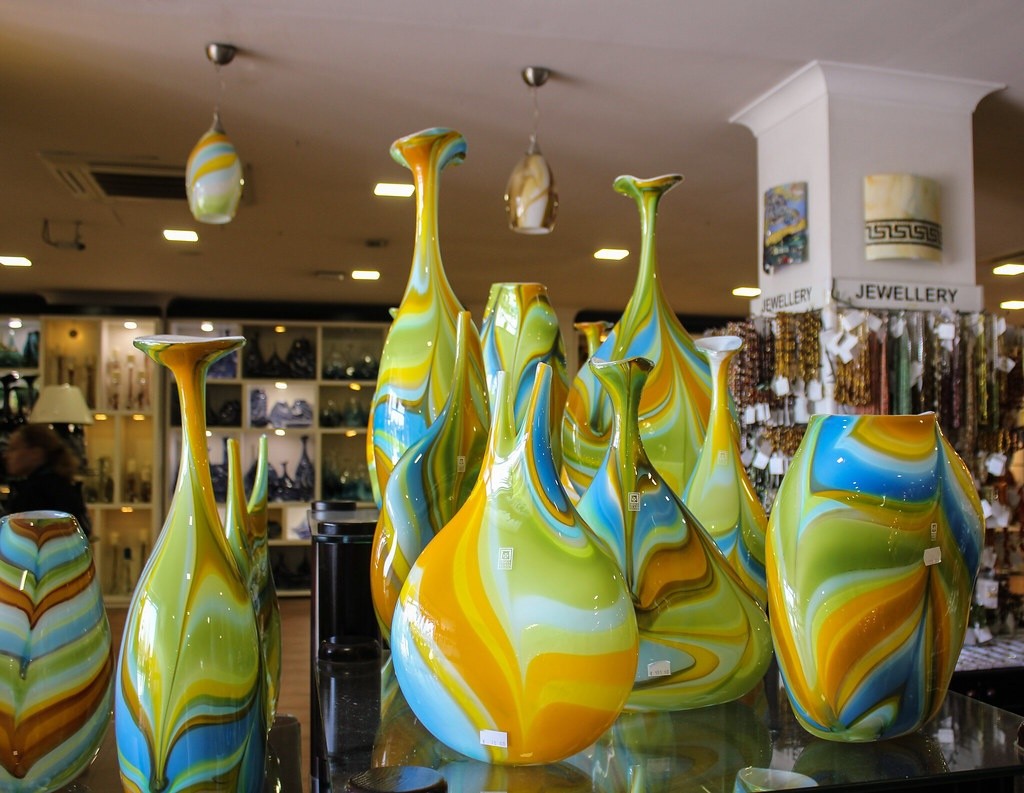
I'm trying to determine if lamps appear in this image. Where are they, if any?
[503,66,561,235]
[184,43,245,225]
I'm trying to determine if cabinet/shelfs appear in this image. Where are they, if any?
[318,322,394,510]
[158,319,320,599]
[1,310,161,609]
[310,520,1024,792]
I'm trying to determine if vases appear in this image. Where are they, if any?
[0,127,988,793]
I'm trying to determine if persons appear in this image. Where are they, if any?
[0,425,92,538]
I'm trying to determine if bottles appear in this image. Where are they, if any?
[0,333,281,793]
[364,127,985,768]
[217,431,314,502]
